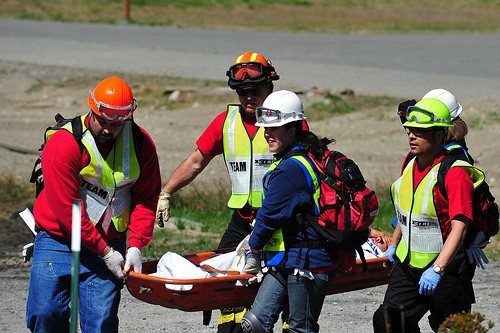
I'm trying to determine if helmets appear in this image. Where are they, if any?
[88,76,138,123]
[225,51,280,88]
[403,97,454,128]
[420,88,463,121]
[254,90,307,127]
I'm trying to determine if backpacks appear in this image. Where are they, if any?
[276,149,379,250]
[30,113,143,199]
[400,147,500,249]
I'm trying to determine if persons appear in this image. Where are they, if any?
[156,51,309,333]
[26,76,162,333]
[148,227,394,290]
[372,88,475,333]
[240,90,342,333]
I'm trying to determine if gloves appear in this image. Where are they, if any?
[419,266,442,295]
[466,231,492,270]
[383,246,396,264]
[97,247,143,280]
[241,253,264,286]
[156,191,176,228]
[22,242,34,263]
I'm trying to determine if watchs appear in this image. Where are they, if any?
[431,264,444,276]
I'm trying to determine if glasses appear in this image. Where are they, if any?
[254,107,296,123]
[406,105,451,124]
[226,62,275,82]
[97,100,133,121]
[236,85,266,96]
